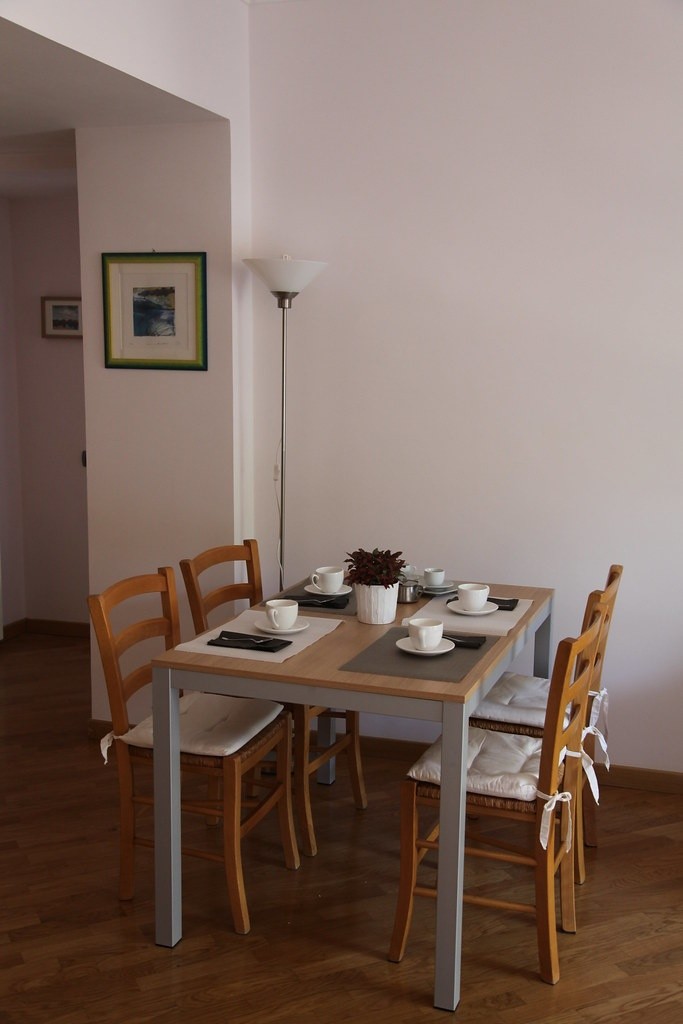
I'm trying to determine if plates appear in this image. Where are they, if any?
[304,585,352,595]
[447,600,498,616]
[396,637,455,656]
[254,616,309,634]
[419,589,458,594]
[420,581,454,591]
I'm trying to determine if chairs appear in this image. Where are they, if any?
[84,539,368,936]
[393,565,624,987]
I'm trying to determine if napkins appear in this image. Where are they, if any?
[206,630,293,655]
[446,593,519,609]
[403,634,487,650]
[281,592,349,609]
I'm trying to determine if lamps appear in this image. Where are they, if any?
[244,254,328,589]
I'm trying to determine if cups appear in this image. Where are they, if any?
[266,599,298,629]
[400,564,416,573]
[458,584,489,611]
[310,566,344,593]
[397,585,425,603]
[408,618,443,650]
[424,568,445,585]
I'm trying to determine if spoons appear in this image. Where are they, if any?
[296,598,335,604]
[222,636,272,643]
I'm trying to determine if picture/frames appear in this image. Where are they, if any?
[36,293,84,337]
[98,250,212,371]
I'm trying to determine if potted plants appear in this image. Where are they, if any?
[344,548,404,624]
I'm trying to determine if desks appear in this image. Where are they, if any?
[149,578,552,1010]
